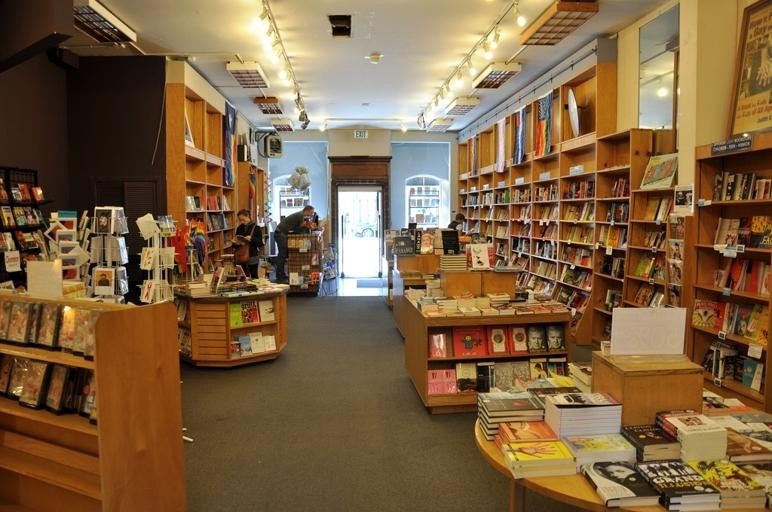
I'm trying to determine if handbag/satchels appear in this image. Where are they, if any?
[234,245,249,262]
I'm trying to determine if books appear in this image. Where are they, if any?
[173,263,283,358]
[384,222,471,301]
[2,193,55,274]
[1,297,132,425]
[476,340,771,511]
[558,176,630,340]
[406,242,569,394]
[47,203,177,304]
[634,167,770,395]
[184,194,234,229]
[460,186,558,293]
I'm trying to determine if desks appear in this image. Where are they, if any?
[384,239,571,414]
[1,293,187,511]
[474,389,772,512]
[173,281,290,367]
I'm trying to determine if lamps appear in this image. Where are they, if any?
[258,0,310,130]
[417,1,525,129]
[656,75,667,96]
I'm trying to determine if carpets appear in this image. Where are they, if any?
[357,279,382,288]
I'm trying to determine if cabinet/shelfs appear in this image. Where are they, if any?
[622,188,674,308]
[458,65,653,350]
[692,128,772,402]
[166,84,264,271]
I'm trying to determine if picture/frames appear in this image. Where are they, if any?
[726,0,772,139]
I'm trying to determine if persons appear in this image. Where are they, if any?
[232,208,264,279]
[262,206,315,284]
